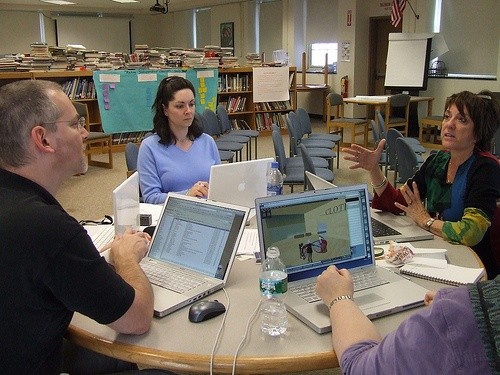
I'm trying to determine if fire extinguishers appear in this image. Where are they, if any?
[340,75,348,104]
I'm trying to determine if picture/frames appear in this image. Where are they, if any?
[220,22,234,57]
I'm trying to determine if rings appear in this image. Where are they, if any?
[357,157,359,162]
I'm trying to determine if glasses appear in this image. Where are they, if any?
[39,117,85,130]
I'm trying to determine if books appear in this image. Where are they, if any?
[218,74,249,92]
[400,257,485,287]
[102,131,151,145]
[230,119,251,131]
[254,101,290,110]
[255,113,288,130]
[0,42,239,72]
[246,52,285,67]
[217,94,246,114]
[59,80,96,99]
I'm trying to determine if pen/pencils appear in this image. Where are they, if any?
[199,183,206,187]
[99,244,112,254]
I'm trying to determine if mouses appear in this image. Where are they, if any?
[188,299,226,323]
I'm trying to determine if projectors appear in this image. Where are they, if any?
[150,6,166,12]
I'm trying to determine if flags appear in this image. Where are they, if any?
[390,0,407,28]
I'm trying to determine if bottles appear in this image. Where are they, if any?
[259,247,289,337]
[267,162,282,197]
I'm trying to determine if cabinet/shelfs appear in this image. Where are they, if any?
[0,67,297,155]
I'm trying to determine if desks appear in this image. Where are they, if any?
[65,204,488,375]
[262,52,331,123]
[341,96,435,143]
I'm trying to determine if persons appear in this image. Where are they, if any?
[0,80,154,375]
[139,76,222,204]
[341,90,500,280]
[314,264,500,375]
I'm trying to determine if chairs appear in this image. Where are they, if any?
[123,93,444,193]
[71,102,113,176]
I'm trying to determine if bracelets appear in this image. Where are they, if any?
[371,175,387,189]
[328,295,353,312]
[424,218,435,232]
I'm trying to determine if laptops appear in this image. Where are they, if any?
[79,158,431,334]
